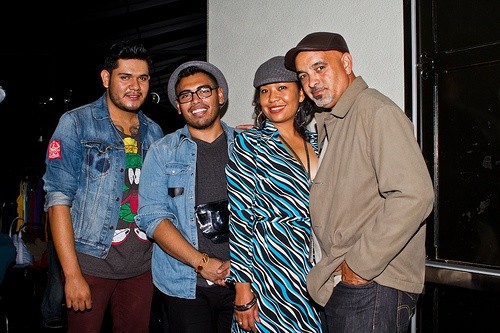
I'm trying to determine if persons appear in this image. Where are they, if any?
[235,31,435,333]
[43,40,164,333]
[225,55,325,333]
[135,61,248,333]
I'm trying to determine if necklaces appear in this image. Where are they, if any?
[278,127,312,183]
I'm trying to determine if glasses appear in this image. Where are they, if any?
[176,85,218,104]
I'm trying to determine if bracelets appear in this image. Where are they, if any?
[194,252,209,273]
[232,292,258,311]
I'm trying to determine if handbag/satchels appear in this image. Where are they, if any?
[9,217,32,264]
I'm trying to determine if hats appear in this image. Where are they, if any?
[167,61,228,110]
[253,56,299,88]
[284,32,350,71]
